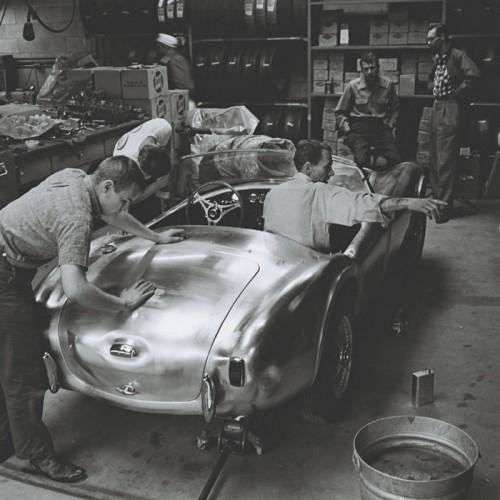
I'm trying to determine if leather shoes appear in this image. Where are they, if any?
[31,456,85,483]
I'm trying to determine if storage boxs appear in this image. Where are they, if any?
[60,64,190,130]
[314,13,439,167]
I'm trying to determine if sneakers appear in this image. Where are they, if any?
[435,209,450,224]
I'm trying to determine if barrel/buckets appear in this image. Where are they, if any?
[352,415,479,500]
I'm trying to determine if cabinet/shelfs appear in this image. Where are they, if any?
[307,0,499,199]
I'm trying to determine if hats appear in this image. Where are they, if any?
[155,33,178,48]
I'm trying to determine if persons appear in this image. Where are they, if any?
[143,29,196,108]
[0,152,194,485]
[332,51,401,175]
[262,136,452,258]
[418,21,481,225]
[111,115,182,226]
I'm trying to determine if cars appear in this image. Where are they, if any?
[30,148,428,435]
